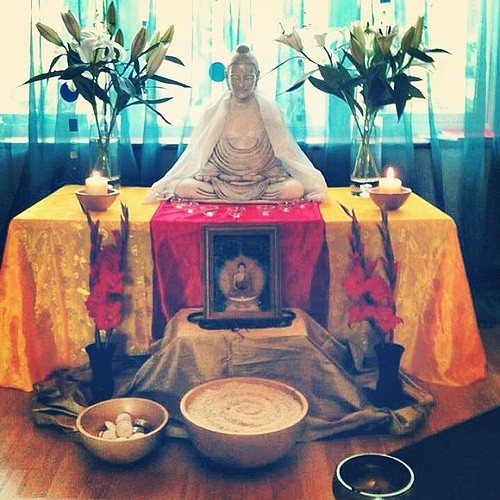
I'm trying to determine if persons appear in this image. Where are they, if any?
[229,262,257,297]
[176,46,303,205]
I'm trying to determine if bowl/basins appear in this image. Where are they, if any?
[369,187,413,211]
[75,189,120,211]
[76,397,169,464]
[333,453,414,500]
[179,375,313,469]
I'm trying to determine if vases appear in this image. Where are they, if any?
[84,342,117,400]
[349,113,383,199]
[374,343,405,410]
[87,114,121,190]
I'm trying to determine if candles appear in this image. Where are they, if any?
[378,166,401,192]
[85,171,107,194]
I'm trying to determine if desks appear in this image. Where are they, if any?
[0,184,488,391]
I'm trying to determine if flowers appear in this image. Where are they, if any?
[79,199,129,353]
[19,1,191,178]
[335,198,403,349]
[268,13,452,181]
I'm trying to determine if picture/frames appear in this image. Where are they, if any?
[201,223,281,326]
